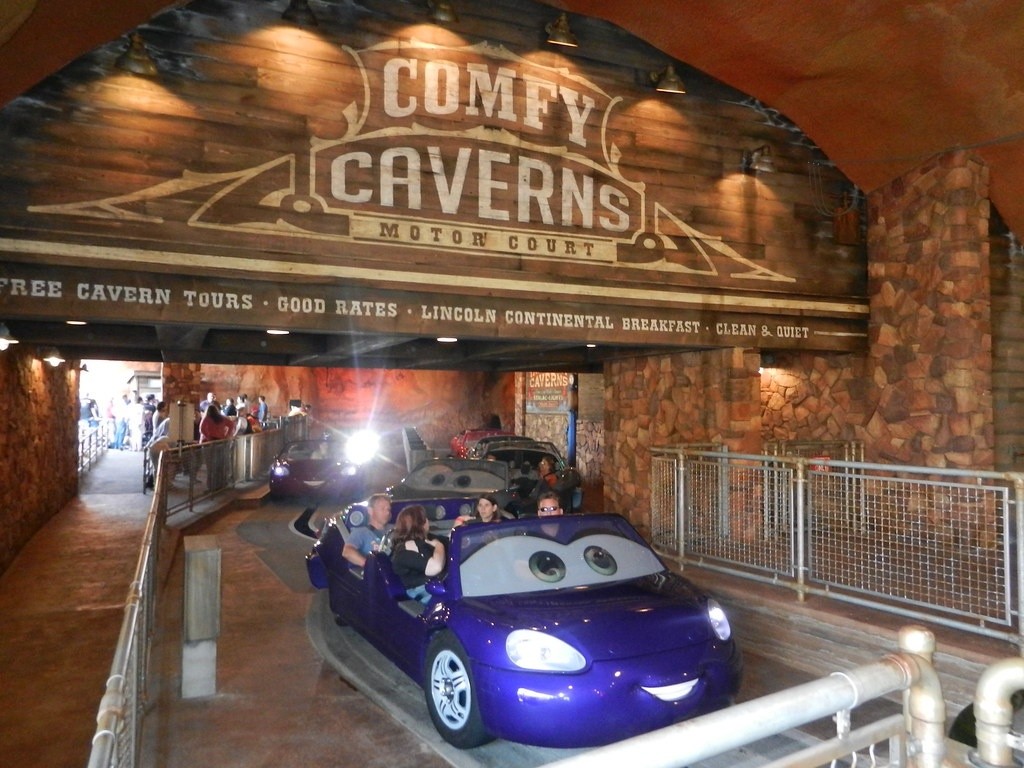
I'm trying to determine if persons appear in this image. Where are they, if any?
[342,494,398,566]
[311,441,335,459]
[286,403,311,418]
[390,504,446,605]
[449,494,502,540]
[538,457,559,486]
[86,389,270,492]
[485,451,497,461]
[535,491,564,518]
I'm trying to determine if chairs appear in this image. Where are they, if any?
[379,528,452,596]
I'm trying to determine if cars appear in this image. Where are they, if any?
[269,424,741,750]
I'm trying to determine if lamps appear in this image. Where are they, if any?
[650,58,686,94]
[282,0,318,26]
[427,0,460,23]
[545,12,578,48]
[746,143,777,175]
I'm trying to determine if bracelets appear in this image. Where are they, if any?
[455,517,465,523]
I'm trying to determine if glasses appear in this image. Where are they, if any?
[539,507,560,512]
